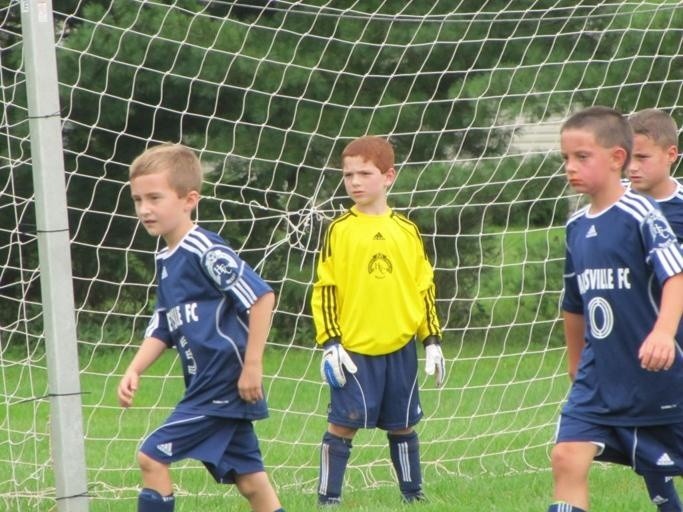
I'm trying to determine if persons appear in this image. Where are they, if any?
[116,139,285,512]
[308,133,447,506]
[546,106,683,511]
[624,108,682,510]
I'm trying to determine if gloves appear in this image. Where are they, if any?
[321,343,358,387]
[425,343,446,385]
